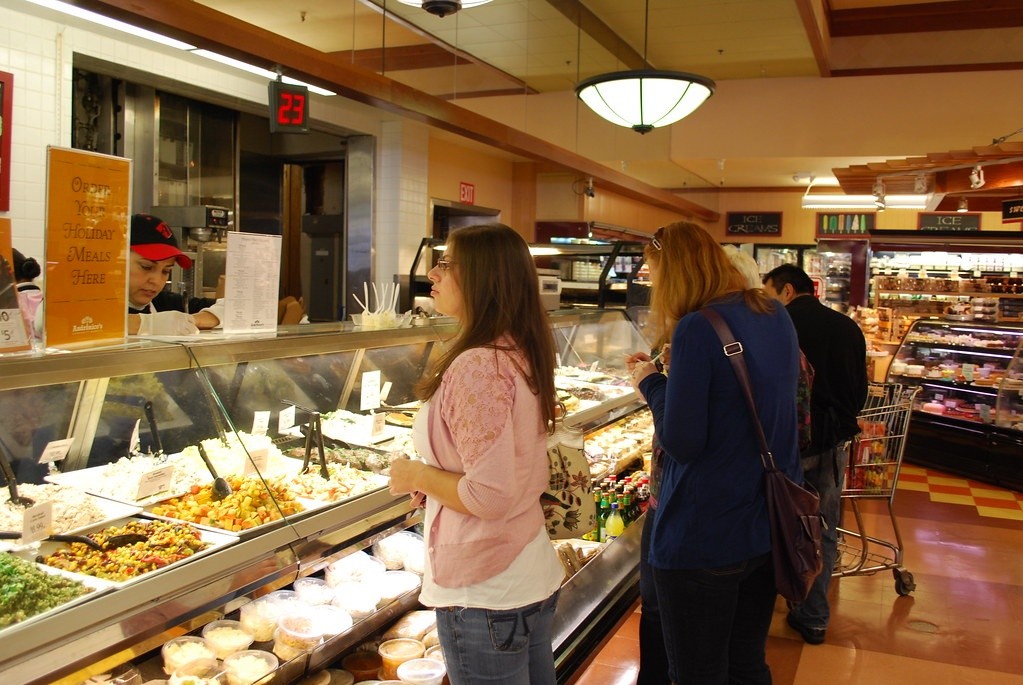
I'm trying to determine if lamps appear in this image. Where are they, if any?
[914,173,929,191]
[968,164,986,189]
[872,177,886,214]
[573,1,717,134]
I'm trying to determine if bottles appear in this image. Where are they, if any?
[582,474,649,543]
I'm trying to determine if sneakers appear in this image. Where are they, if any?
[785,612,827,645]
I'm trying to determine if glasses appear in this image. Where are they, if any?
[437,257,453,269]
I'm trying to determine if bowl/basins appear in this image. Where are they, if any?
[94,517,453,684]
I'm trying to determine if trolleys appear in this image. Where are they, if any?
[824,380,923,596]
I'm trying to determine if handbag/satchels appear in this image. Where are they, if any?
[539,400,598,540]
[767,469,824,604]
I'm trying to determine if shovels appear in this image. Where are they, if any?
[198,442,232,501]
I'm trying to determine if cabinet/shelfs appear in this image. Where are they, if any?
[866,287,1023,397]
[1,307,661,685]
[884,318,1023,489]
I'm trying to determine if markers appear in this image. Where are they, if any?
[182,290,189,313]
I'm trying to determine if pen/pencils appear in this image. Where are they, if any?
[652,349,669,363]
[622,353,642,362]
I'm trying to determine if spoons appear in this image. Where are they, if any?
[198,443,232,501]
[352,281,400,319]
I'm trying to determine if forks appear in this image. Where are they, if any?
[298,411,331,480]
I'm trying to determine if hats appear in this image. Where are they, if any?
[130,214,192,270]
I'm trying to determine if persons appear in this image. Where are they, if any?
[126,211,228,337]
[386,222,565,685]
[10,246,46,341]
[628,220,802,685]
[763,263,869,645]
